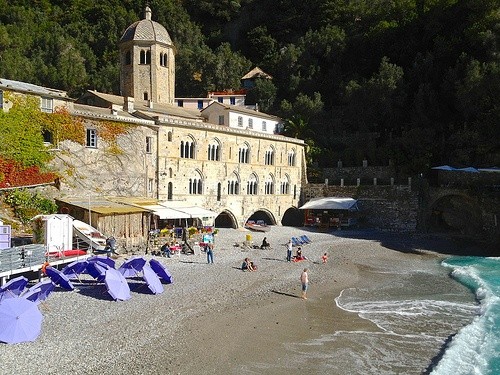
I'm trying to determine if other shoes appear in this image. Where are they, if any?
[301,296,307,299]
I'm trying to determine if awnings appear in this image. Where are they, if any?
[299,197,358,210]
[140,200,218,219]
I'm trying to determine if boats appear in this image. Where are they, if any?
[73,219,107,250]
[257,220,270,232]
[246,221,262,231]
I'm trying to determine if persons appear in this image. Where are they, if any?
[297,247,303,258]
[301,268,309,300]
[242,258,253,272]
[105,236,120,257]
[285,240,292,261]
[161,243,174,258]
[322,253,327,262]
[263,237,269,247]
[207,241,213,264]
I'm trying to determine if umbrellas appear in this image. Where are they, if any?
[0,256,173,344]
